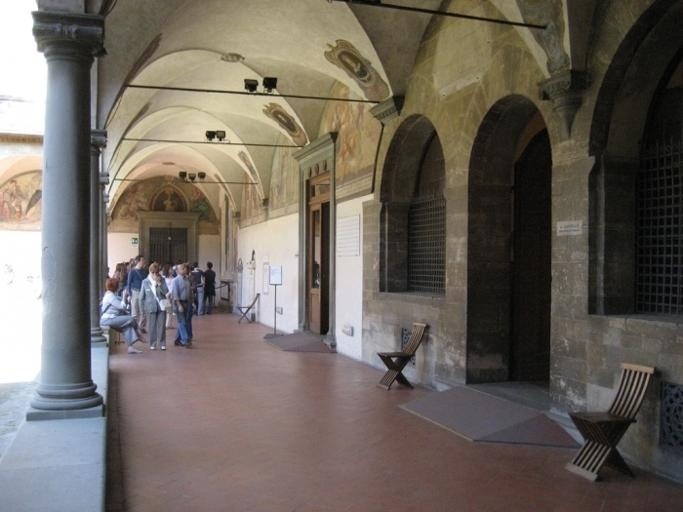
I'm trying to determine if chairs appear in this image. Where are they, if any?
[376,321,428,391]
[564,361,654,482]
[236,294,260,323]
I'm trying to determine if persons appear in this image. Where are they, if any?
[194,262,216,315]
[168,263,194,347]
[137,263,170,351]
[97,278,148,354]
[97,254,206,341]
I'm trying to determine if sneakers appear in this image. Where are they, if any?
[126,340,194,353]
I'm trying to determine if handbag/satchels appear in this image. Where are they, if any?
[159,298,173,312]
